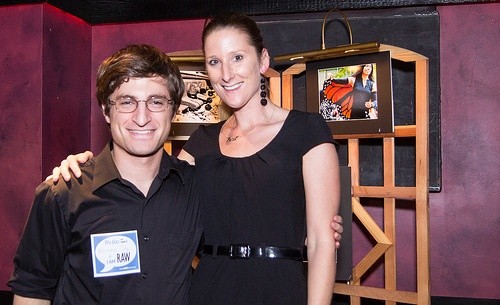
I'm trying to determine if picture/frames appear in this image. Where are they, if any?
[168,62,232,142]
[306,50,395,136]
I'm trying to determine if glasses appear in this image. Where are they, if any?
[107,96,174,113]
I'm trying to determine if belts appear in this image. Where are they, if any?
[203,243,303,261]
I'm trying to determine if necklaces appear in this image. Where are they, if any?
[221,103,276,143]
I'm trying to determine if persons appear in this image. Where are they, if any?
[6,43,343,305]
[46,14,340,305]
[327,64,378,119]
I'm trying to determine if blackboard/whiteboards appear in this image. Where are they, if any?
[255,12,442,189]
